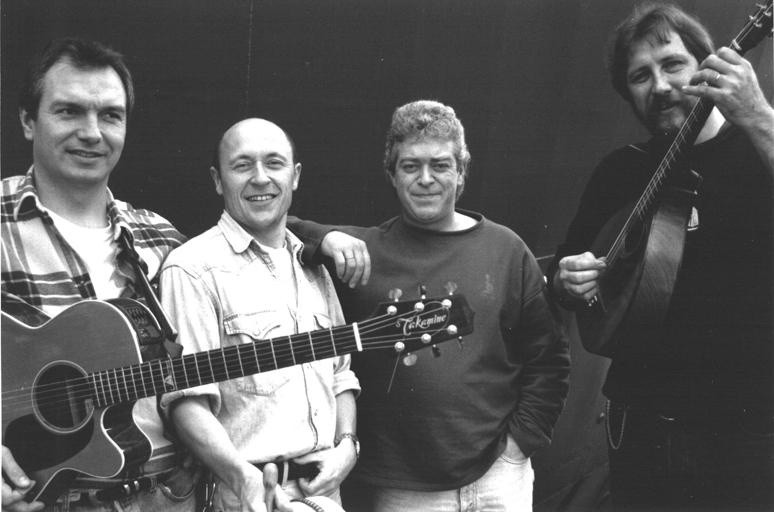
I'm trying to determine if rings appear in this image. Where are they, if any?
[708,74,721,86]
[345,256,354,260]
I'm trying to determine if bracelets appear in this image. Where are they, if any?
[332,433,361,460]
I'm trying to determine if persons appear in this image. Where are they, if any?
[142,113,369,512]
[278,96,573,512]
[543,1,774,511]
[1,30,216,512]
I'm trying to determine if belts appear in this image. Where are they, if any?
[250,454,318,486]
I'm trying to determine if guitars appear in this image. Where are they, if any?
[0,279,476,508]
